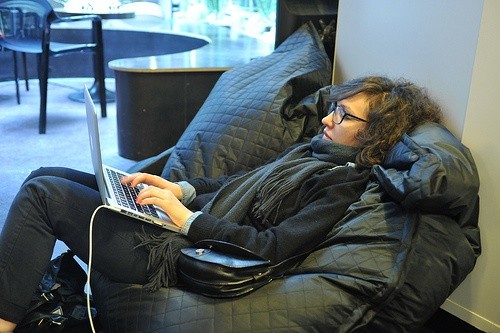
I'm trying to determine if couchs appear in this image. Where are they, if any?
[88,21,482,333]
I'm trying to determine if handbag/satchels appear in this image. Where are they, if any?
[176,238,311,298]
[14,248,98,333]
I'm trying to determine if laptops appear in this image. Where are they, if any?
[84,83,182,233]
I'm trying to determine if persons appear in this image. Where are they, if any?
[0,75,442,333]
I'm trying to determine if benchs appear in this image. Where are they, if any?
[53,15,276,163]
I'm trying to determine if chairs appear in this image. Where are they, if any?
[0,0,109,133]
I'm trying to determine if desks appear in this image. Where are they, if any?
[54,7,135,103]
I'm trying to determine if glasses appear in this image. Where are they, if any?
[328,101,369,125]
